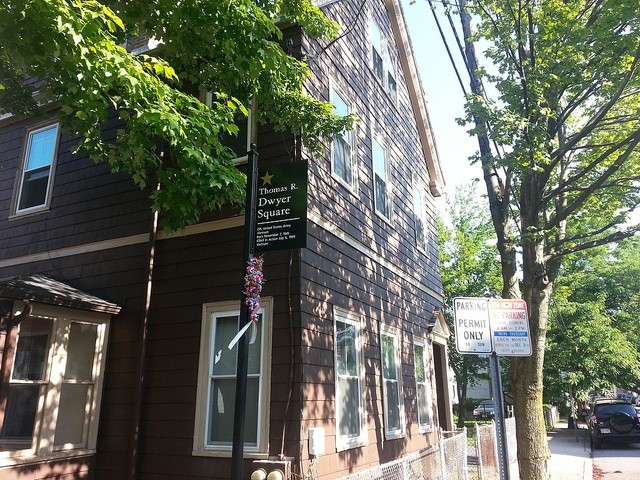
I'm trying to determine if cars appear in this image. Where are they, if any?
[473,400,495,421]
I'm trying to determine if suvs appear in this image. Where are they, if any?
[588,398,640,450]
